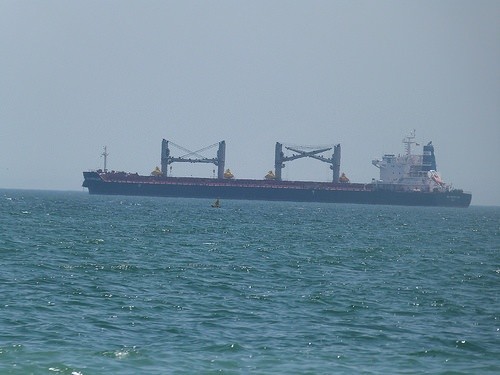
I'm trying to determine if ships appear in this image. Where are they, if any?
[81,131,471,208]
